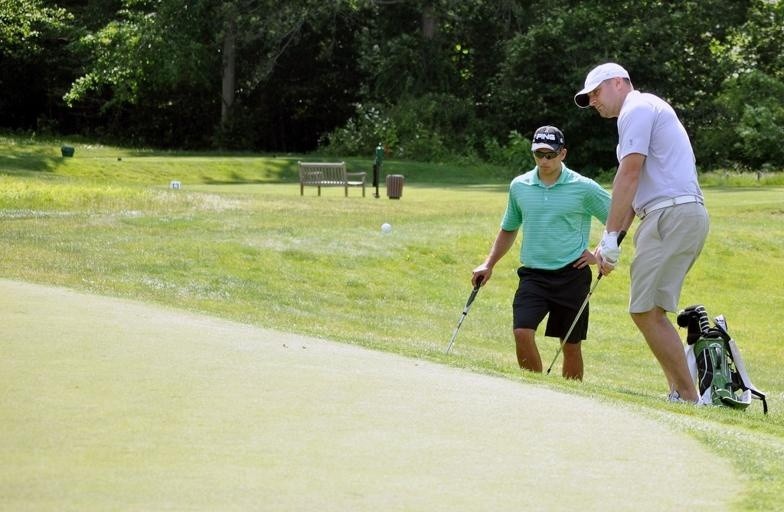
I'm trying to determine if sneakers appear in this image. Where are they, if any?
[669,391,703,405]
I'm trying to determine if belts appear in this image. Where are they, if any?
[639,196,703,220]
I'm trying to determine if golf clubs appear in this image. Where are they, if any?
[714,314,727,335]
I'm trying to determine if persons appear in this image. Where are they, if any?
[470,126,611,381]
[571,64,709,405]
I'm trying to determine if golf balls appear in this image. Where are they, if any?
[381,224,391,232]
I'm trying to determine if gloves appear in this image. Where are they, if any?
[600,231,619,266]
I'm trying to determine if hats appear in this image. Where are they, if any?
[574,62,631,108]
[530,126,564,151]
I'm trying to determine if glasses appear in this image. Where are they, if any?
[534,151,560,159]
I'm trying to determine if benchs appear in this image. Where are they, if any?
[298,161,367,198]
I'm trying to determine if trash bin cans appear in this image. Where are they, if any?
[386,174,404,199]
